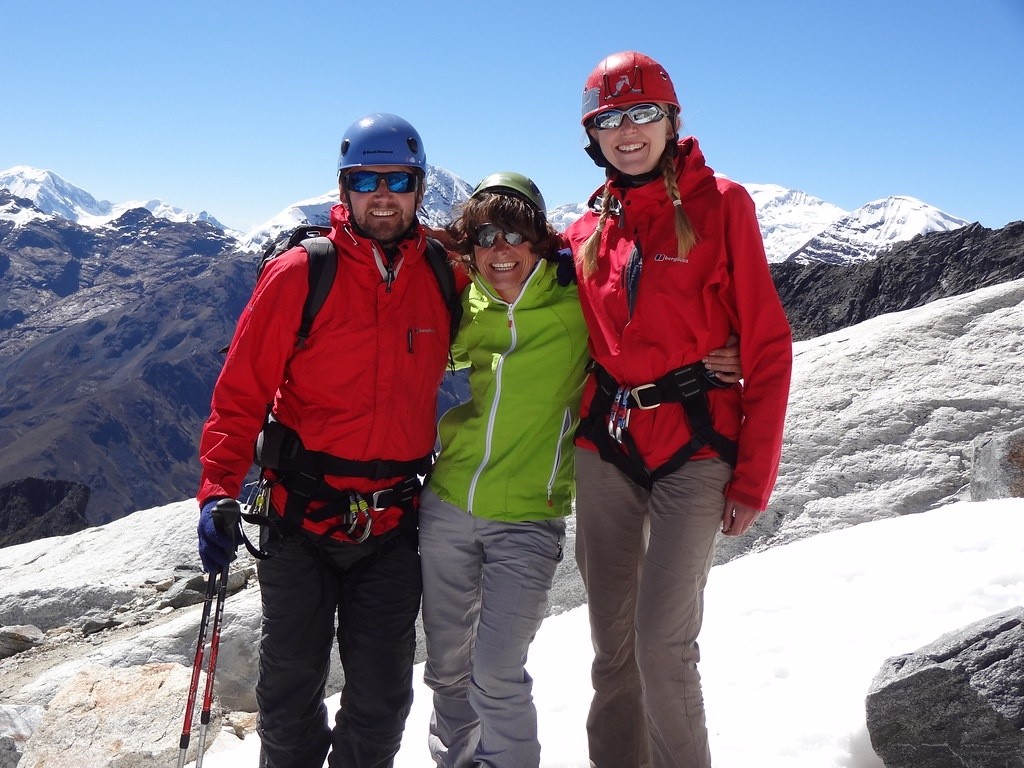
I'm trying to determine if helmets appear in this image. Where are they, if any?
[471,171,547,219]
[338,112,426,177]
[581,50,682,128]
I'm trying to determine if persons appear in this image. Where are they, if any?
[421,51,792,768]
[198,112,578,768]
[419,172,741,768]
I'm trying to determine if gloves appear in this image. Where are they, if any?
[199,497,239,573]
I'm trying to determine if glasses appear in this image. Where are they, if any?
[471,221,527,247]
[345,170,419,192]
[588,103,671,129]
